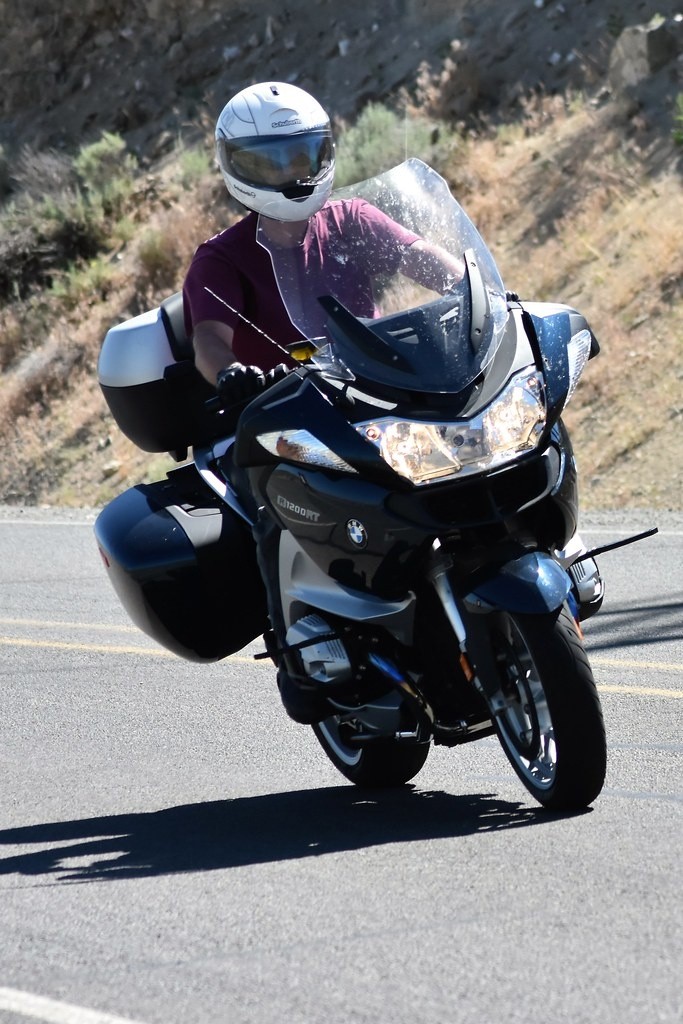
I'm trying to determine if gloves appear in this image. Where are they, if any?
[507,291,521,301]
[215,364,266,415]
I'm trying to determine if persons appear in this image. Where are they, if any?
[184,81,518,670]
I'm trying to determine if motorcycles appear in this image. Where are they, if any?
[91,158,661,816]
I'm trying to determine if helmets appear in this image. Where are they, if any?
[214,80,337,221]
